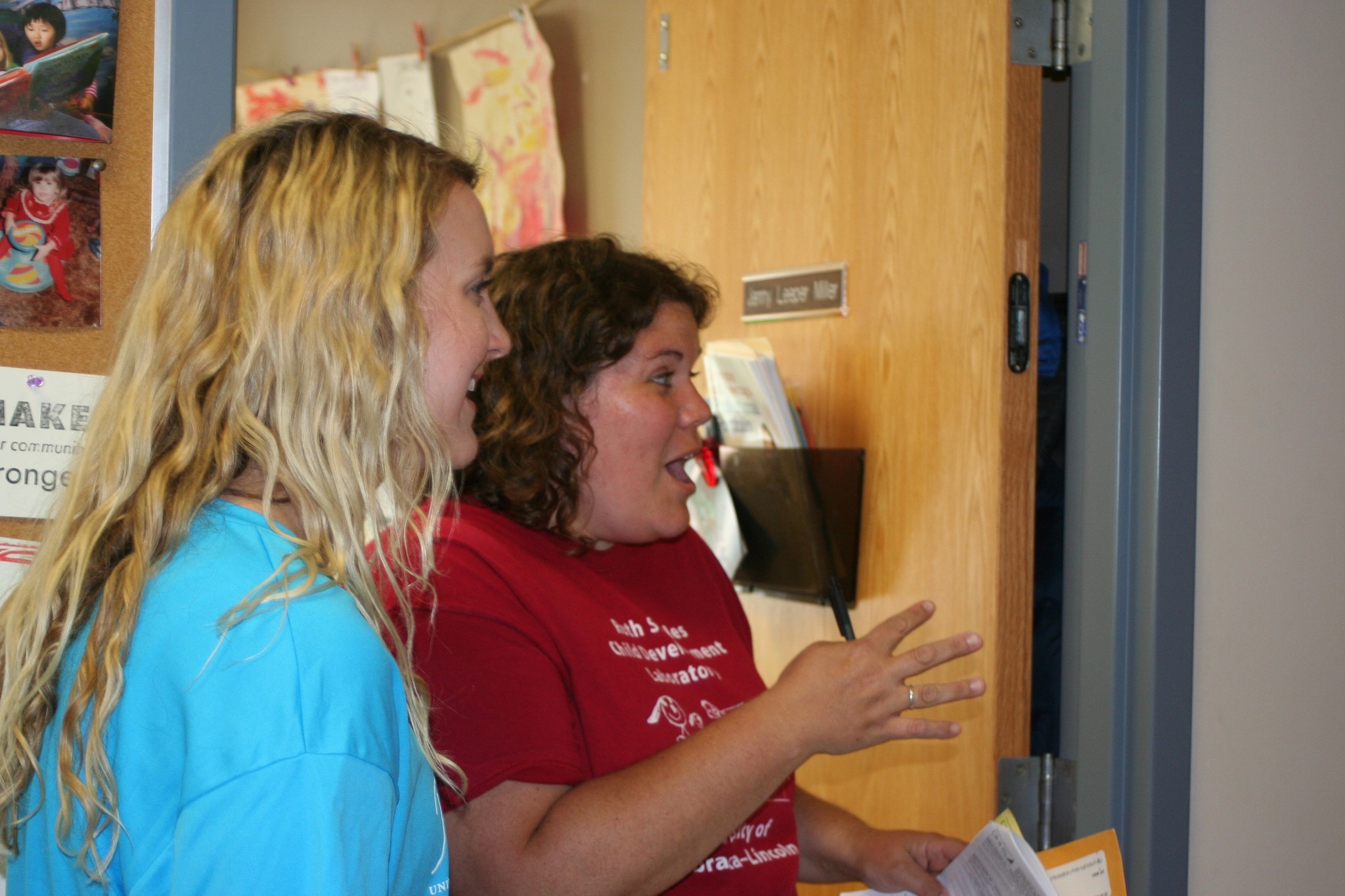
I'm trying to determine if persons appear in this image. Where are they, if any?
[0,102,516,896]
[356,228,989,896]
[19,3,113,143]
[0,161,78,304]
[0,29,102,142]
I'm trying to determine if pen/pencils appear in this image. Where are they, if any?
[826,581,858,645]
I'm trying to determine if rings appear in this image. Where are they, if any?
[906,683,916,712]
